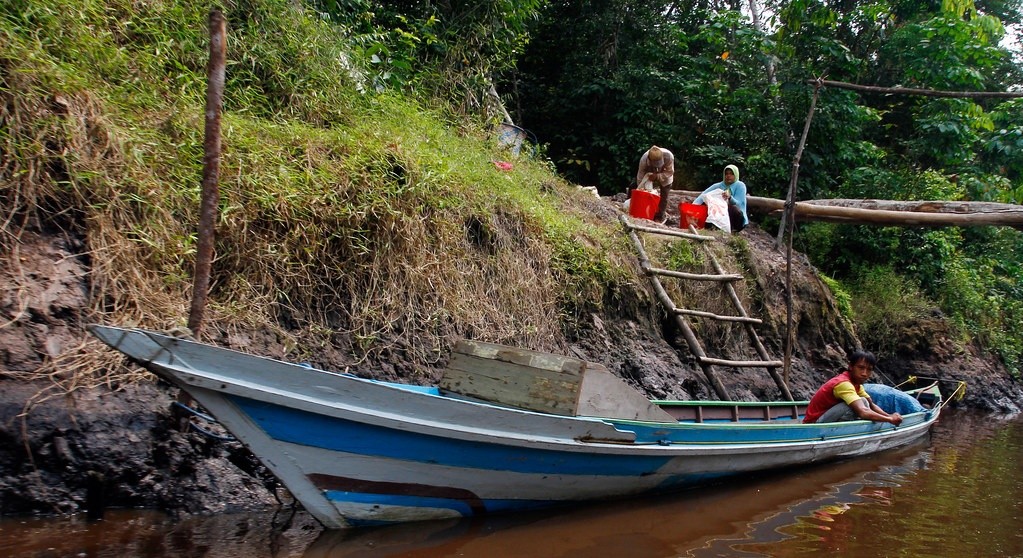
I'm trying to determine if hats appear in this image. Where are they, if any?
[649,147,664,167]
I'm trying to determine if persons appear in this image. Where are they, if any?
[627,145,674,224]
[803,349,903,427]
[692,165,749,231]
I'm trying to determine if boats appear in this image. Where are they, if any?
[84,323,943,529]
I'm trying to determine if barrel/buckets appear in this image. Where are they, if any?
[495,122,528,159]
[677,202,708,231]
[630,189,661,221]
